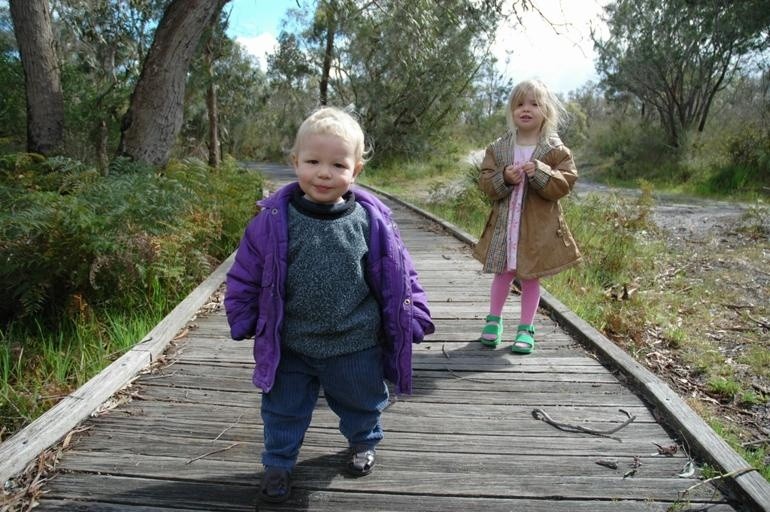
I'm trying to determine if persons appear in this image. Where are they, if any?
[472,78,582,356]
[224,106,436,504]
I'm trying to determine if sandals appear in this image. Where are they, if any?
[480,315,503,346]
[511,324,535,354]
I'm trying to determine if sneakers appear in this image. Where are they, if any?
[345,448,377,475]
[259,465,293,503]
